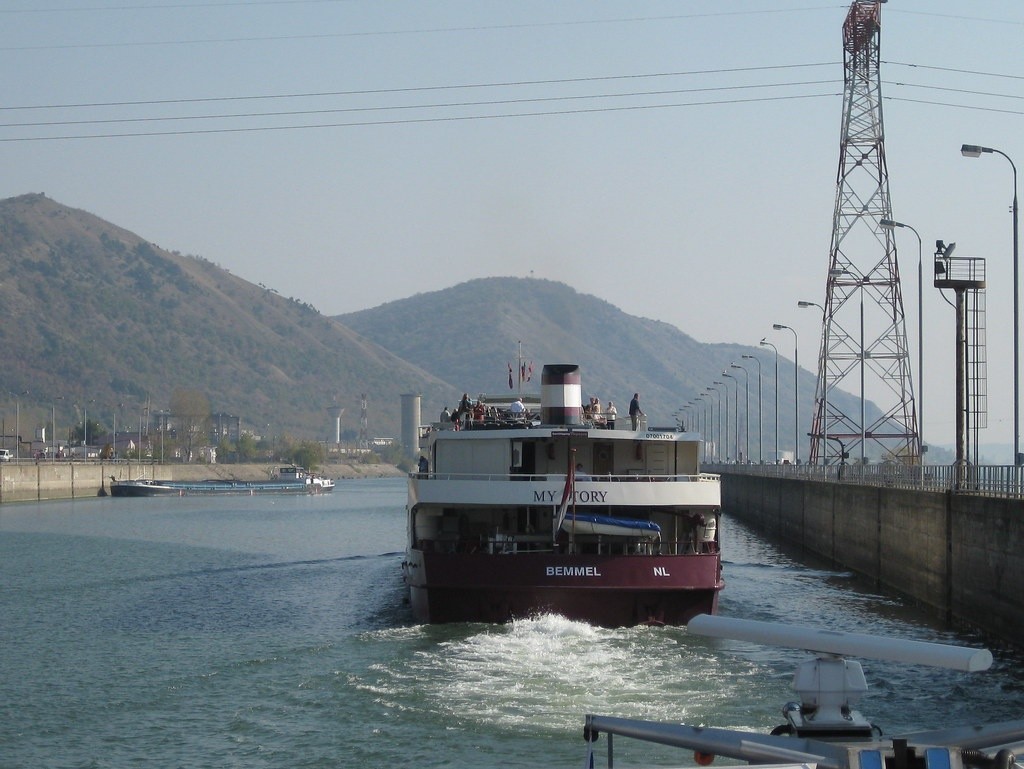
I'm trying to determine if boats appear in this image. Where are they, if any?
[554,511,661,537]
[397,340,726,627]
[111,461,336,497]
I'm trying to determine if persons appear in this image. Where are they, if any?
[585,397,607,429]
[573,463,589,481]
[418,456,428,478]
[629,393,644,431]
[606,402,617,430]
[440,393,530,422]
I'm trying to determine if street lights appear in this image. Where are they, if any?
[961,144,1019,496]
[129,407,170,462]
[730,364,749,465]
[721,372,738,463]
[773,323,800,479]
[742,355,762,467]
[673,387,721,464]
[798,301,827,479]
[9,390,28,462]
[43,396,64,462]
[105,403,124,460]
[713,382,730,463]
[880,219,923,489]
[75,399,96,462]
[760,341,777,475]
[829,269,864,484]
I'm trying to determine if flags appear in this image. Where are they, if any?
[522,360,532,383]
[555,455,574,541]
[507,361,514,389]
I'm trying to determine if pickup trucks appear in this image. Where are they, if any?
[0,449,14,462]
